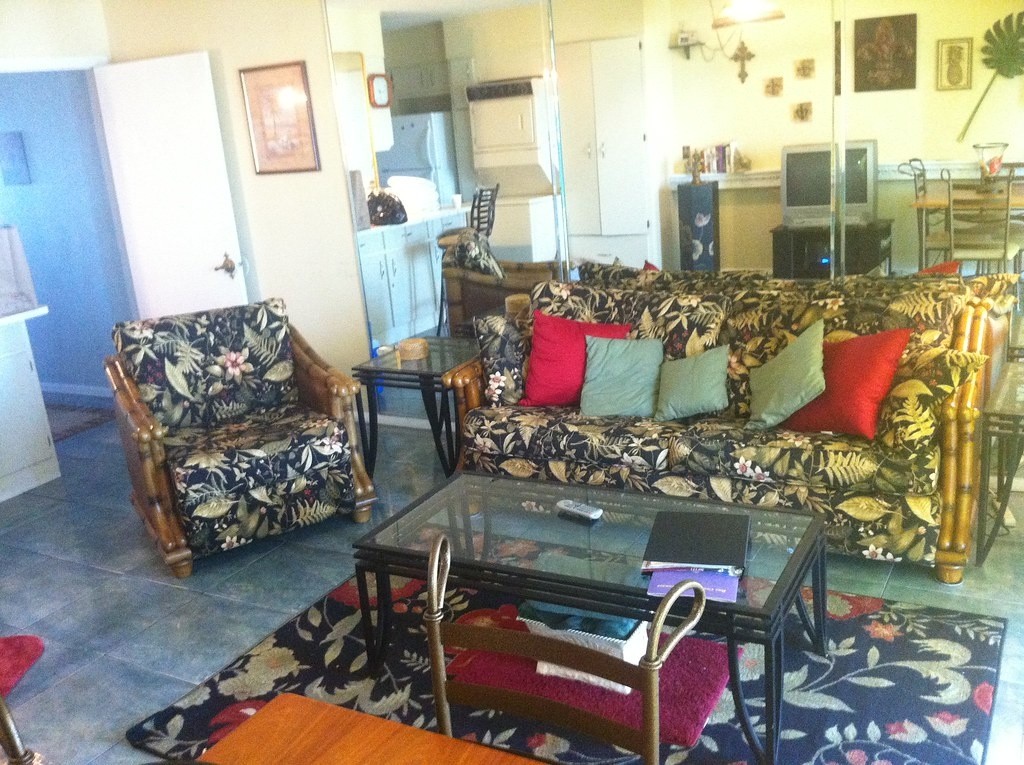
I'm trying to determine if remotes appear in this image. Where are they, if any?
[557,500,603,518]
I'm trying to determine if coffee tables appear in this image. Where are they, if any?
[352,470,829,765]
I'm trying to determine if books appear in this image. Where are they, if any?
[640,512,750,603]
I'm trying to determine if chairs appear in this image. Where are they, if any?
[897,158,1024,274]
[422,534,706,764]
[438,227,568,337]
[104,297,379,578]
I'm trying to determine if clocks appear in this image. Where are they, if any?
[368,74,392,108]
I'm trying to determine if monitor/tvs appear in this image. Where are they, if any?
[782,140,878,227]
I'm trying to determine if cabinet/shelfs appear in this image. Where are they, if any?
[0,320,62,501]
[770,218,895,277]
[555,39,650,269]
[357,213,466,349]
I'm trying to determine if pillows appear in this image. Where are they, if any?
[654,343,731,421]
[922,262,960,274]
[782,327,916,440]
[519,310,633,406]
[744,318,826,431]
[580,334,663,417]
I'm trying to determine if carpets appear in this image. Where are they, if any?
[126,522,1009,765]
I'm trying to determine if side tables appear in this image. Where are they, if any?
[976,361,1024,567]
[352,337,480,478]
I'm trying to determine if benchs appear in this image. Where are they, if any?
[442,261,1018,587]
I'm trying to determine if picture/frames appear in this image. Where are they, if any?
[239,60,321,175]
[937,37,973,90]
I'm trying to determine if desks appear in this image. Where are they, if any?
[196,694,540,765]
[908,184,1024,210]
